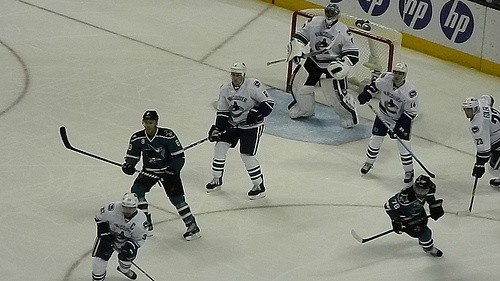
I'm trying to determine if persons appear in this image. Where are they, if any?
[384,174,444,257]
[205,63,274,200]
[357,63,417,184]
[287,3,360,128]
[123,110,201,241]
[91,193,148,281]
[462,95,500,188]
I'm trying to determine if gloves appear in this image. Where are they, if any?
[357,91,412,141]
[208,106,263,142]
[472,164,485,178]
[123,158,181,179]
[429,199,444,221]
[392,217,403,234]
[98,221,141,260]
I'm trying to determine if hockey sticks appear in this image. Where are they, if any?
[166,119,250,155]
[366,100,457,181]
[59,125,165,182]
[266,30,341,66]
[350,215,433,244]
[456,173,479,216]
[113,244,155,281]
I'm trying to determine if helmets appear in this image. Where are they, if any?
[413,175,430,195]
[142,110,158,125]
[462,97,479,114]
[230,61,246,78]
[392,63,408,79]
[325,4,340,19]
[121,193,138,213]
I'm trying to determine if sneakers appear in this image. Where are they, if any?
[489,178,500,188]
[426,247,443,257]
[404,169,414,183]
[147,222,153,236]
[247,185,266,200]
[361,162,373,174]
[206,178,222,191]
[117,265,137,280]
[183,226,201,241]
